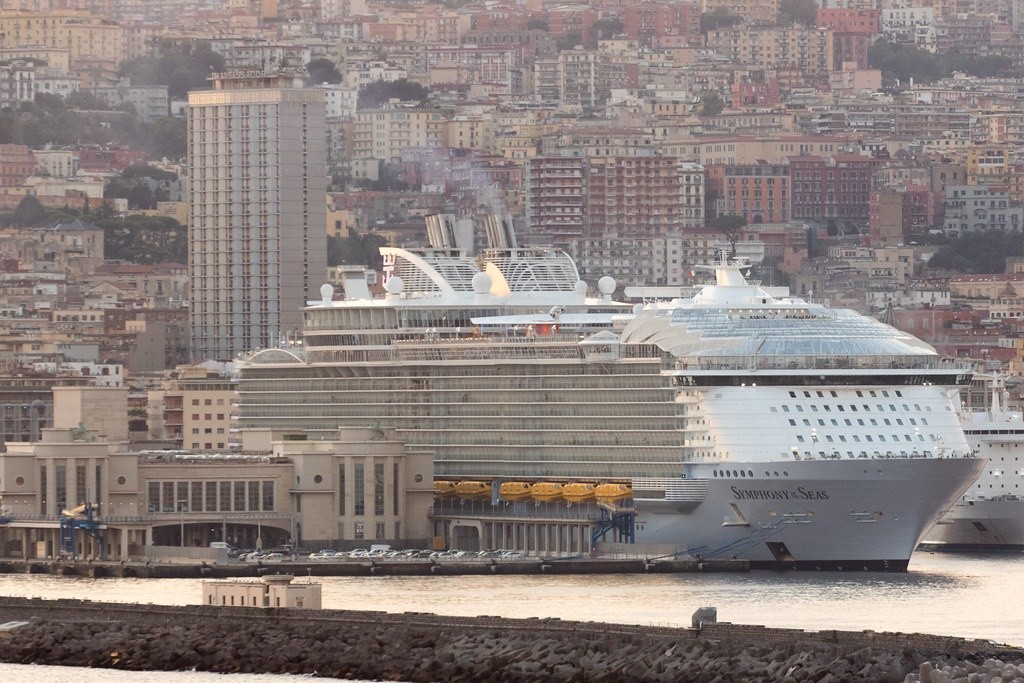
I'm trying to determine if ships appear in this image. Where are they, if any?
[0,241,990,589]
[915,369,1024,554]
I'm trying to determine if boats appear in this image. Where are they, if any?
[595,483,633,503]
[433,479,459,501]
[455,480,492,500]
[529,482,562,500]
[560,482,597,502]
[500,481,531,502]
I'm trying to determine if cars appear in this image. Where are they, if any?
[211,541,523,562]
